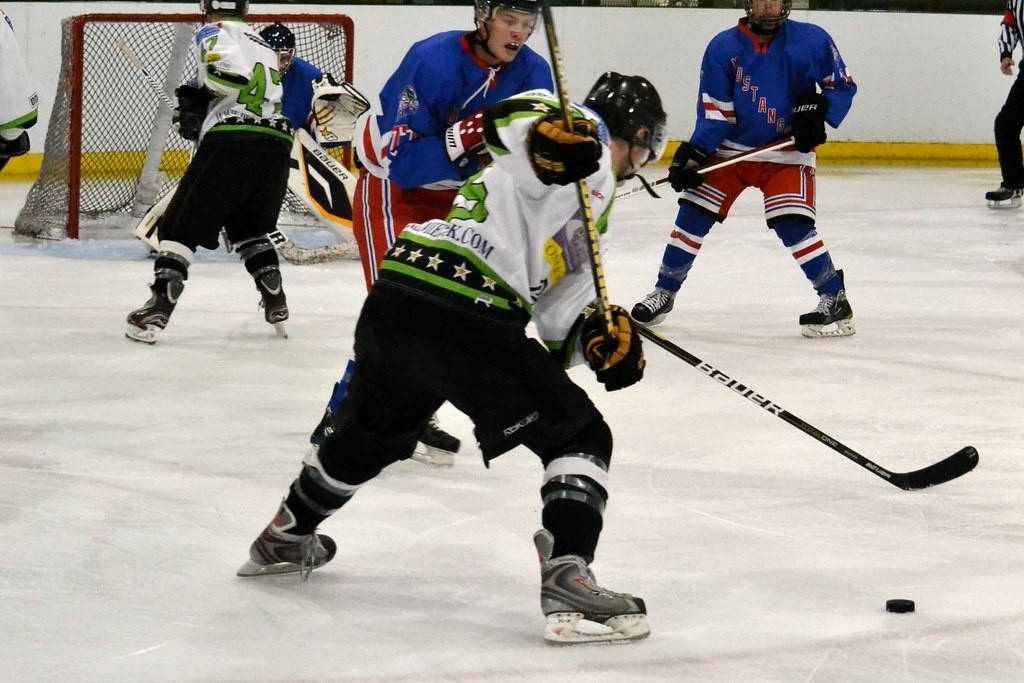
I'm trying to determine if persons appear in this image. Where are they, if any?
[985,0,1024,208]
[629,1,859,339]
[256,19,371,246]
[123,0,295,345]
[350,0,554,470]
[234,72,671,645]
[0,8,38,171]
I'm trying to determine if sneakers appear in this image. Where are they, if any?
[409,415,461,468]
[300,382,340,466]
[799,269,857,337]
[236,497,336,578]
[125,280,184,345]
[259,274,291,339]
[984,184,1024,209]
[632,288,686,327]
[533,530,651,643]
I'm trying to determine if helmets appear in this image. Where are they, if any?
[744,0,792,36]
[201,0,249,20]
[585,71,670,165]
[259,24,296,78]
[474,0,539,25]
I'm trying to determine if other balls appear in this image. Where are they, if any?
[886,598,915,614]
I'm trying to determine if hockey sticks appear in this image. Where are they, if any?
[540,2,618,343]
[586,300,980,492]
[611,135,797,202]
[267,224,356,266]
[114,39,236,256]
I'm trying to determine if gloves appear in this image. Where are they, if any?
[174,86,209,141]
[786,94,827,151]
[524,115,602,187]
[579,304,645,392]
[667,141,714,193]
